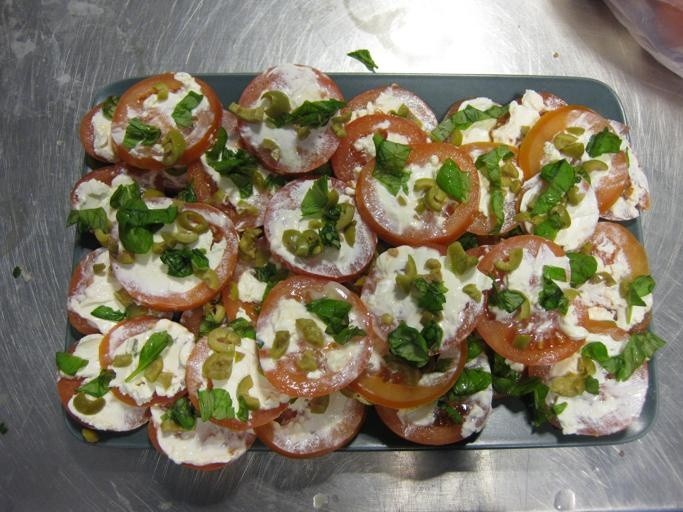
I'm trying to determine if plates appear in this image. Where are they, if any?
[61,72,658,453]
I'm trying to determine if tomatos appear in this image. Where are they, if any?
[57,62,654,472]
